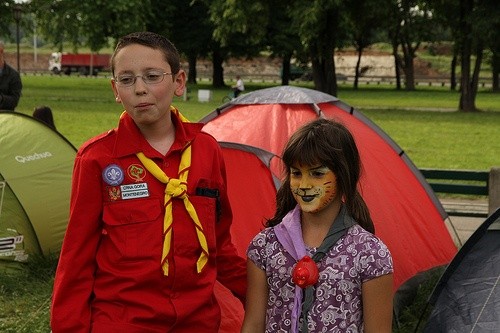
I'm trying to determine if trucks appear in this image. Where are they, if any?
[48,52,110,75]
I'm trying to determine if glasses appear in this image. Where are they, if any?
[112,70,176,85]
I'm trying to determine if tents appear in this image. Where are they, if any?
[0,106,79,279]
[197,86,463,333]
[413,207,500,333]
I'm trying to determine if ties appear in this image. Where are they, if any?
[120,104,211,278]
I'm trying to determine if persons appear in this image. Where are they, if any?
[0,40,57,130]
[50,31,247,333]
[232,75,244,98]
[240,118,394,333]
